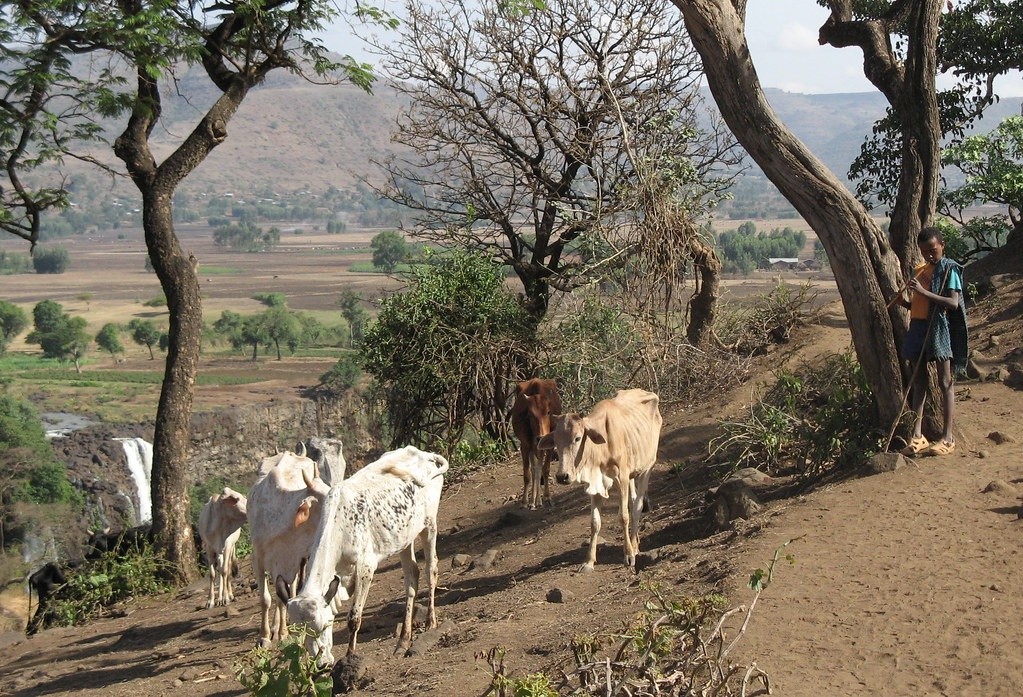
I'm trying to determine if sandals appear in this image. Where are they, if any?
[902,433,929,456]
[928,438,955,456]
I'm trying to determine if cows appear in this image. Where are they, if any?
[28,378,662,673]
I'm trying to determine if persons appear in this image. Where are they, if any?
[892,227,961,456]
